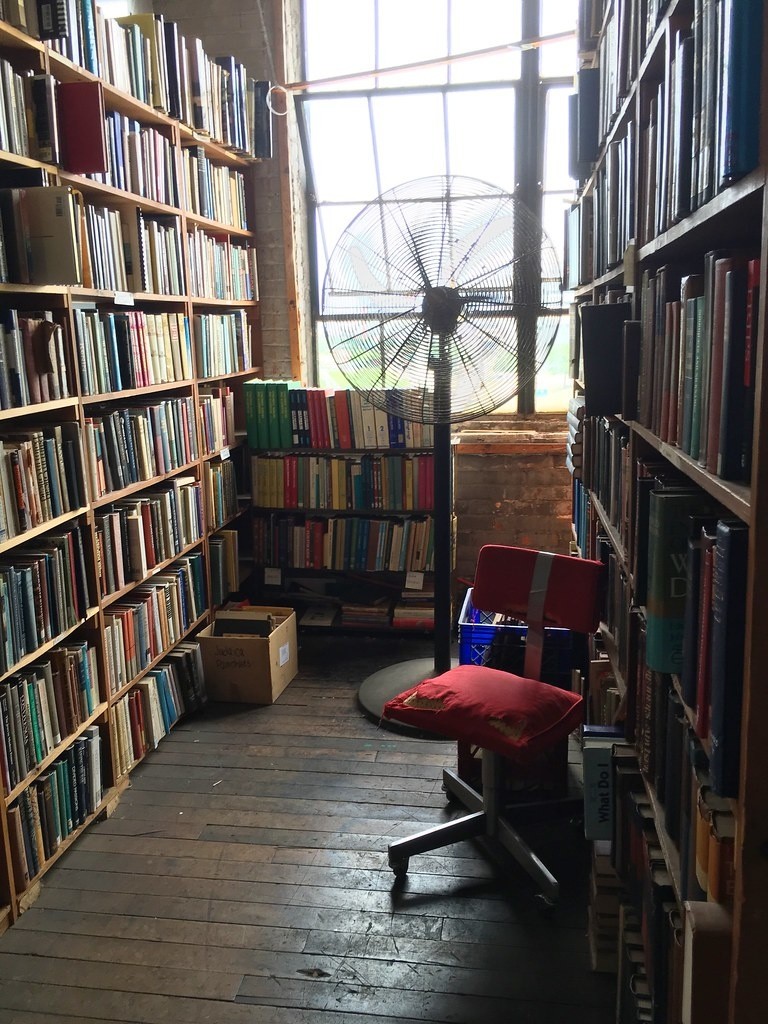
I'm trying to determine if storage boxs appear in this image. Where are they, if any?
[457,586,587,688]
[197,606,296,705]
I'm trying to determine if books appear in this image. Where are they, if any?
[263,576,435,629]
[250,449,435,513]
[0,0,288,889]
[252,514,436,572]
[558,0,768,1024]
[480,611,527,673]
[241,376,435,448]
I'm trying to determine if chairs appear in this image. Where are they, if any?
[383,541,603,915]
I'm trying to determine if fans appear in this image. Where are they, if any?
[323,175,566,739]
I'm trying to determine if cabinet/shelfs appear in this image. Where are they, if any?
[566,0,768,1024]
[0,0,453,940]
